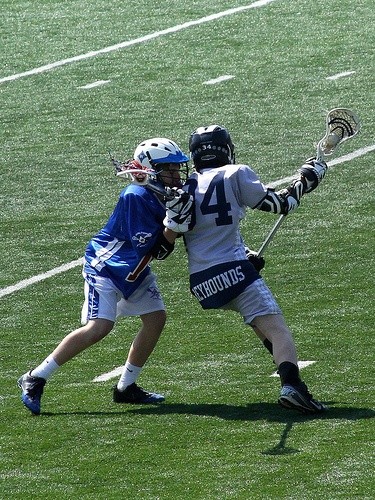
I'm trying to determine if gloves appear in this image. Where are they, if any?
[161,186,194,234]
[245,246,266,275]
[298,156,328,194]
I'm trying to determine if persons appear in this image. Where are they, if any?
[181,126,328,414]
[18,139,193,416]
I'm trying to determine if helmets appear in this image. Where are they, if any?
[131,137,190,200]
[187,125,237,171]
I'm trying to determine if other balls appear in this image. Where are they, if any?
[327,133,339,145]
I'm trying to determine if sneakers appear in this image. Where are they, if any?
[277,378,325,413]
[111,382,165,404]
[17,368,47,415]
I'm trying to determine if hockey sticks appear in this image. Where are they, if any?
[114,159,175,201]
[257,108,360,258]
